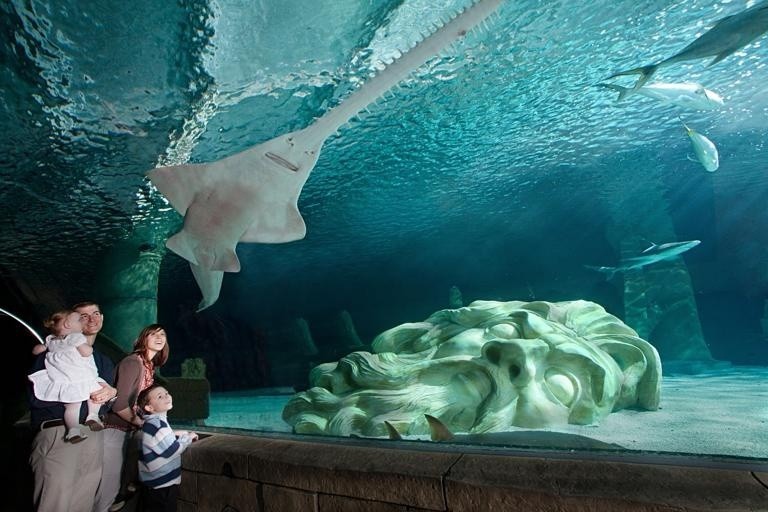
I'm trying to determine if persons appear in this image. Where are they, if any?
[134,385,199,512]
[282,297,663,437]
[29,300,117,512]
[28,310,107,444]
[92,323,169,512]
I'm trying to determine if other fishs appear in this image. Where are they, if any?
[584,240,702,282]
[349,414,628,452]
[600,0,768,94]
[145,0,509,315]
[592,81,725,111]
[678,115,719,172]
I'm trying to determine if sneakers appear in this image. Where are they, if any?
[85,415,106,431]
[65,428,89,444]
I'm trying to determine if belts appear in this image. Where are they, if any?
[43,416,104,428]
